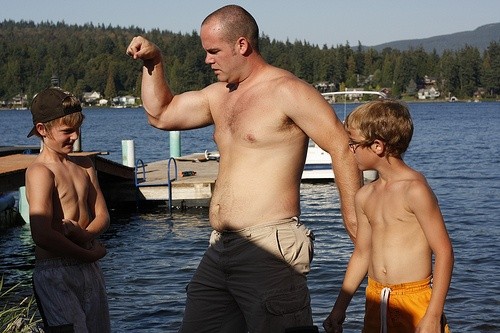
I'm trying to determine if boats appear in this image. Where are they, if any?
[299,137,335,180]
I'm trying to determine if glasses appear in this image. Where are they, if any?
[348,138,373,152]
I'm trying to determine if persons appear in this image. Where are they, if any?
[321,98,454,333]
[125,4,366,333]
[26,85,113,333]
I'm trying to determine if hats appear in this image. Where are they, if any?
[27,86,82,140]
